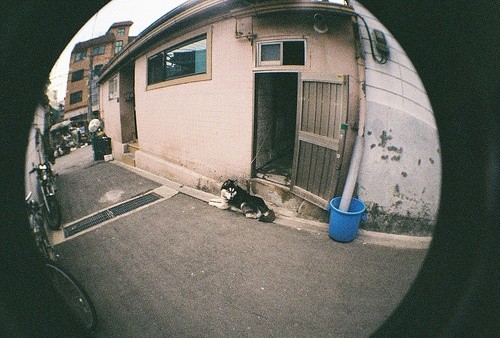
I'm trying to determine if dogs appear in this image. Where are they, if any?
[209,179,276,223]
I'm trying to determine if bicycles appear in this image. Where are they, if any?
[23,192,96,333]
[28,161,63,231]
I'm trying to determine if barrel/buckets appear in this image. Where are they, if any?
[329,196,366,244]
